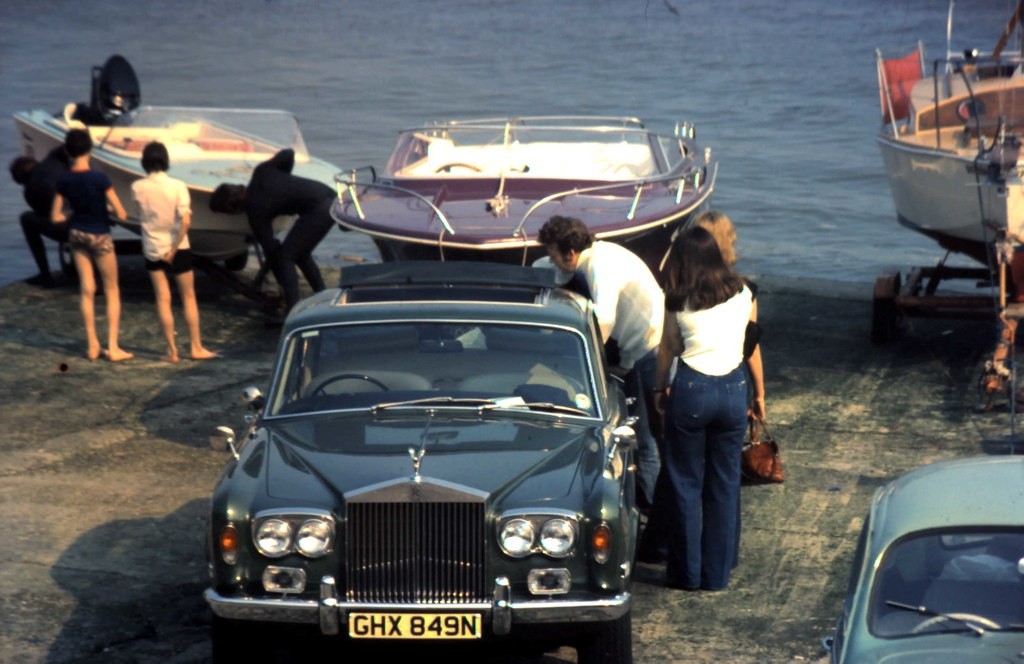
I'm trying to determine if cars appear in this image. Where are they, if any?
[821,449,1024,663]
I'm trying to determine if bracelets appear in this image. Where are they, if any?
[651,386,665,394]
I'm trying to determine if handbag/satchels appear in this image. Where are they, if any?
[740,413,782,486]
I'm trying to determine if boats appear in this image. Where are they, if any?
[330,114,719,299]
[875,1,1024,310]
[12,104,353,267]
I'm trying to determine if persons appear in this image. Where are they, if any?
[538,215,667,563]
[129,141,222,361]
[647,212,767,595]
[51,130,133,362]
[986,234,1024,393]
[210,148,337,317]
[10,147,70,289]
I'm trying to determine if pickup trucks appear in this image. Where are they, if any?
[205,257,651,664]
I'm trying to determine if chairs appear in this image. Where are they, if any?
[460,372,584,401]
[305,370,432,396]
[921,554,1024,626]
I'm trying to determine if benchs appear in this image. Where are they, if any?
[315,328,583,394]
